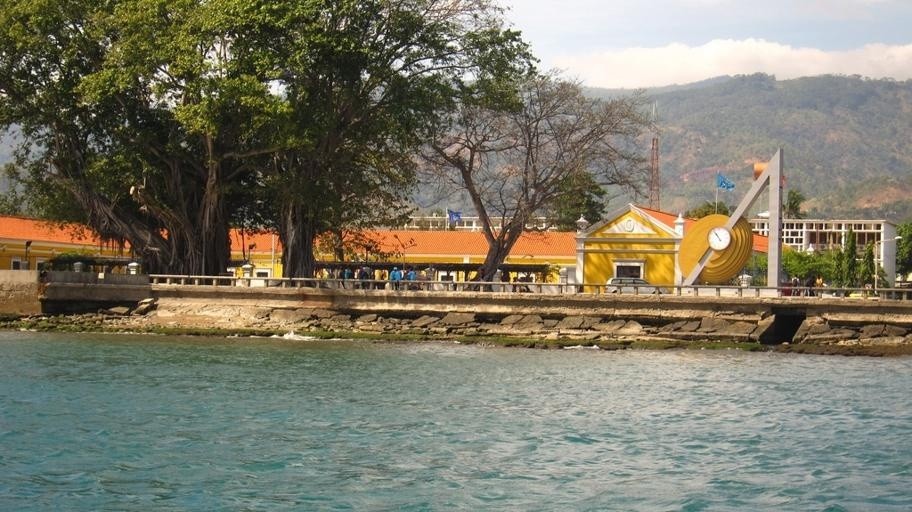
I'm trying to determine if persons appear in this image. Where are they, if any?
[336,266,427,290]
[790,275,800,295]
[803,278,814,296]
[815,275,822,287]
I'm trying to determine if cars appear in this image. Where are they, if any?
[604,277,669,295]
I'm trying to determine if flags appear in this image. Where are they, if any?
[447,208,463,223]
[717,173,736,192]
